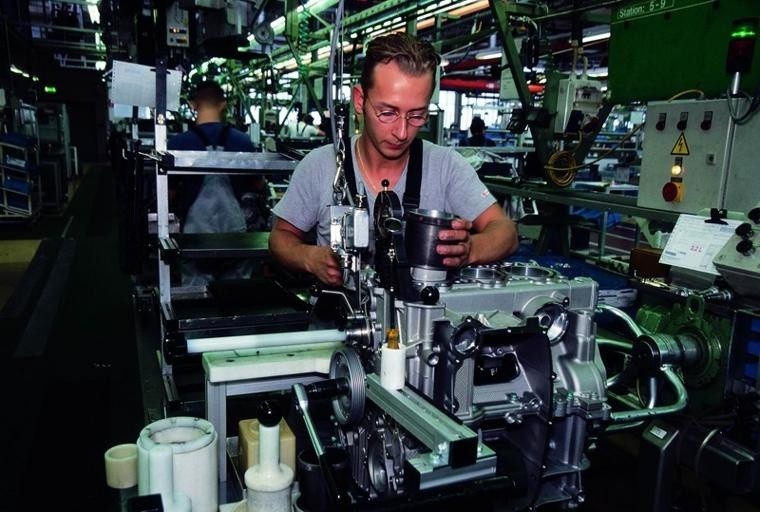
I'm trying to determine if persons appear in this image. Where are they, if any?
[168,81,269,234]
[459,117,496,147]
[268,32,520,287]
[289,115,326,138]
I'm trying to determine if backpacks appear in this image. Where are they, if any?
[178,126,263,288]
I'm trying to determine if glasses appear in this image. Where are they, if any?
[365,94,430,128]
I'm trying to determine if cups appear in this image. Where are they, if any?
[105,443,138,489]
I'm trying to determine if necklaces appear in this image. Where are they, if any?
[357,135,379,193]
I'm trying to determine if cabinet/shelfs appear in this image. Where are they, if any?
[155,149,321,416]
